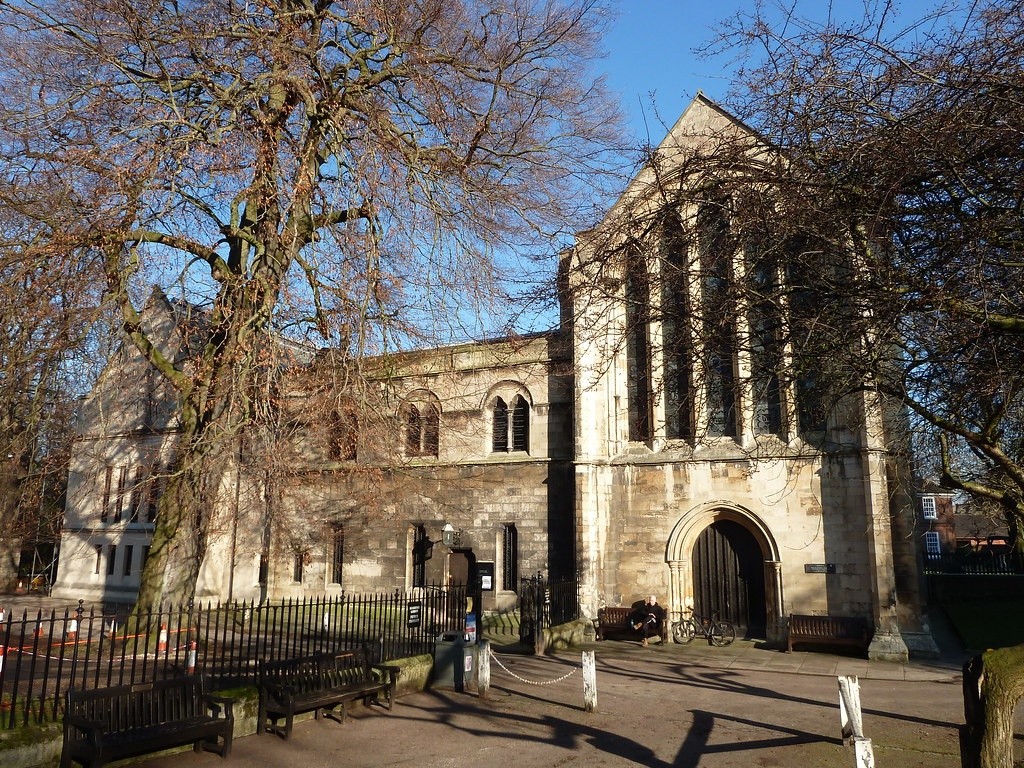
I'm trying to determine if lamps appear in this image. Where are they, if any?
[441,522,461,549]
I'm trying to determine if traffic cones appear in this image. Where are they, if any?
[67,610,78,641]
[33,621,44,637]
[158,623,167,651]
[187,642,197,672]
[0,607,3,633]
[0,644,6,679]
[108,613,118,639]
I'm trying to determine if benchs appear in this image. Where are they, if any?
[59,672,237,768]
[785,612,868,653]
[258,643,401,741]
[599,606,668,645]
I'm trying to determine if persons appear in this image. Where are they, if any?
[633,596,663,647]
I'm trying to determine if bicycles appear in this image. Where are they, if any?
[672,607,736,648]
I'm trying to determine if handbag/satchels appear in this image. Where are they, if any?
[627,618,634,629]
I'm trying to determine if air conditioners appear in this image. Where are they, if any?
[922,496,937,520]
[926,532,941,559]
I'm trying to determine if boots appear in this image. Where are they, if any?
[642,638,648,647]
[633,622,642,630]
